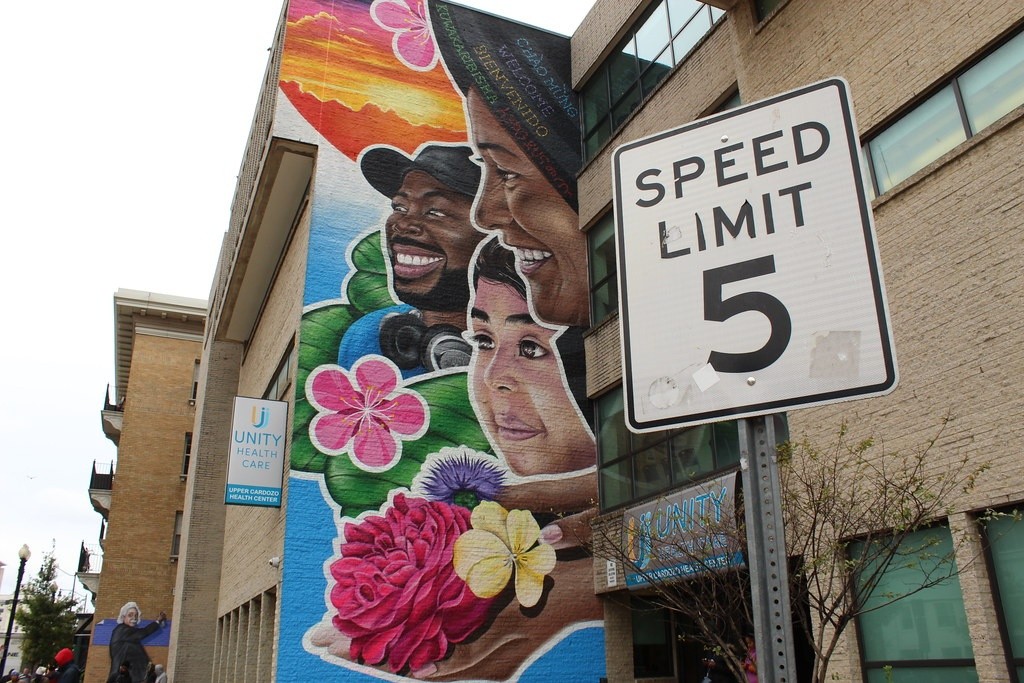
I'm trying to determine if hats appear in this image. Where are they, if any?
[54,648,74,666]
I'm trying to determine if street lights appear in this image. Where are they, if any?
[0,544,31,680]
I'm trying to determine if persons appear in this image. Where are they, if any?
[701,626,740,683]
[105,661,133,683]
[1,648,85,683]
[742,634,758,683]
[145,662,166,683]
